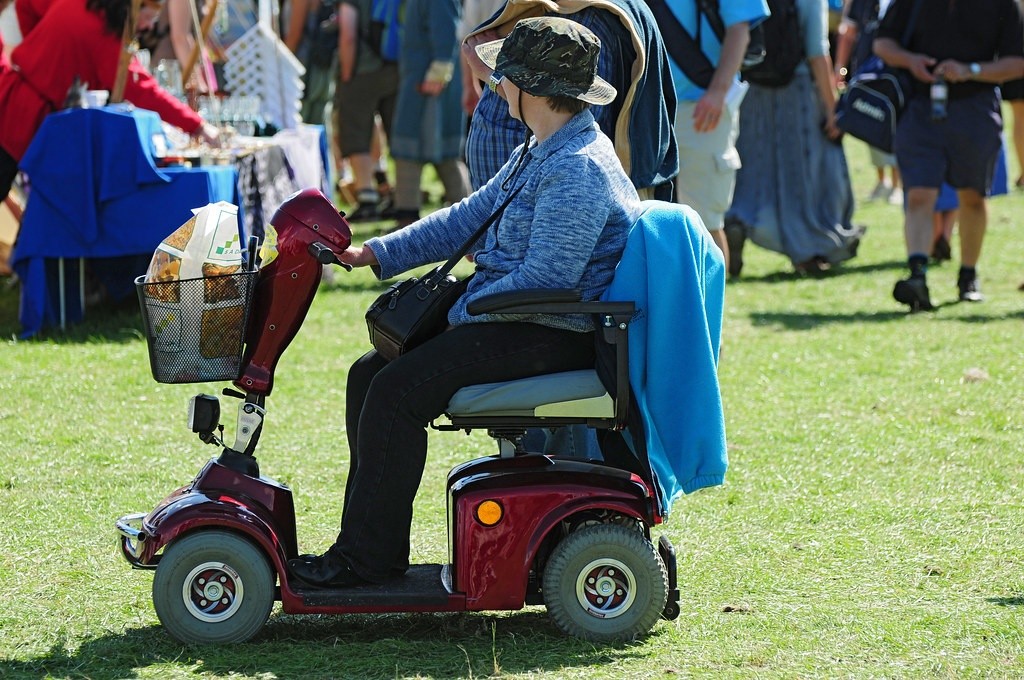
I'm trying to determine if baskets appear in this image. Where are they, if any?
[134,264,259,383]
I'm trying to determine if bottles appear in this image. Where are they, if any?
[930,67,948,122]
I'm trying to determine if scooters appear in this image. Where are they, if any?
[114,186,681,649]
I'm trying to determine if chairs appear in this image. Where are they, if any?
[447,201,707,433]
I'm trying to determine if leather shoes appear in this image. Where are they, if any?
[287,552,407,587]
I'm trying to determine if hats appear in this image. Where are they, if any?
[475,17,619,106]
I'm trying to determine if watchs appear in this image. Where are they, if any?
[488,70,505,94]
[969,60,981,81]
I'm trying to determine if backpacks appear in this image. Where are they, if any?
[739,0,805,90]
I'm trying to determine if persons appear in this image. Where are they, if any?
[295,16,643,590]
[0,0,1024,311]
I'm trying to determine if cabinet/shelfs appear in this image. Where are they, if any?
[8,101,331,340]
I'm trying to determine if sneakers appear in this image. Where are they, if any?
[893,277,932,311]
[956,276,985,303]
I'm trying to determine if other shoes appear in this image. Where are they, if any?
[335,174,421,234]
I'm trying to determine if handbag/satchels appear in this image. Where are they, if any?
[145,200,254,384]
[366,264,459,363]
[831,55,915,153]
